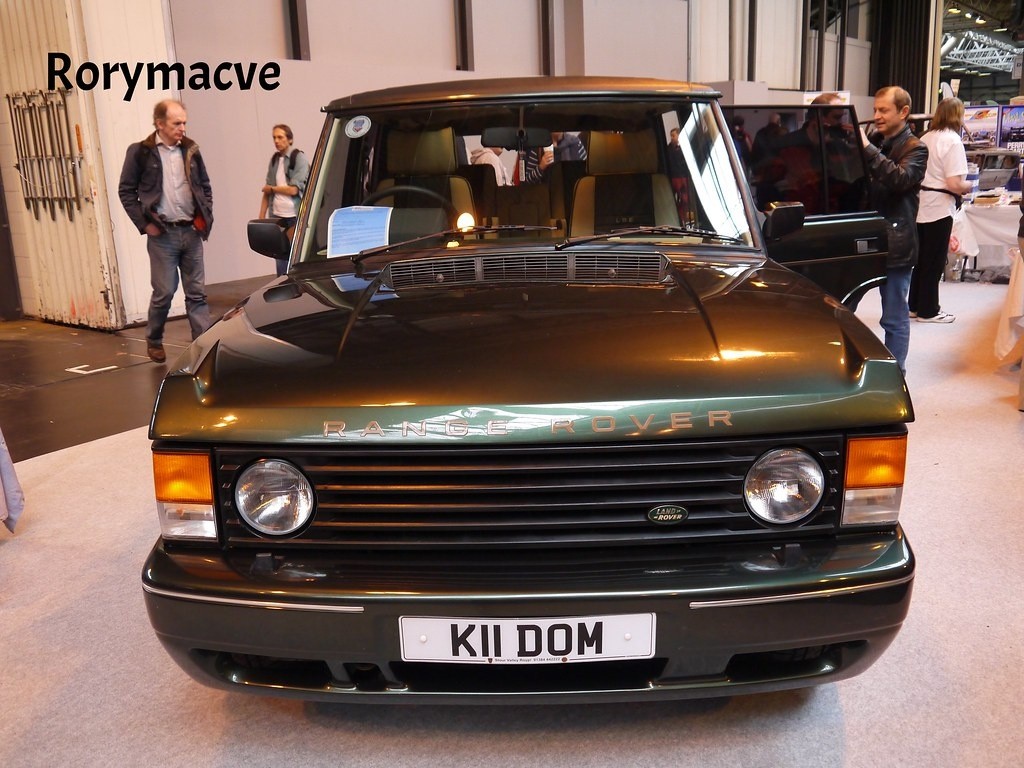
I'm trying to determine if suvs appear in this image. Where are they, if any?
[136,74,917,710]
[853,111,1018,191]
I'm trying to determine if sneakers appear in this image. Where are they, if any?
[908,311,955,323]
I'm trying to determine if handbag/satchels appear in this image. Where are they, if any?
[947,202,980,257]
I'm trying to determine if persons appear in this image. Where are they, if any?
[668,86,929,379]
[117,100,215,363]
[470,131,589,187]
[908,97,974,324]
[259,124,311,278]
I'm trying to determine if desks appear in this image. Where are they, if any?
[943,191,1022,282]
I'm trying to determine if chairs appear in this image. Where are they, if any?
[372,127,479,240]
[569,131,683,237]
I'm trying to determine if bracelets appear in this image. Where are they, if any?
[271,185,274,193]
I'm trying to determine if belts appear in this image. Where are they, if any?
[163,220,195,228]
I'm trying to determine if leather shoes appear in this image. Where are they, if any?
[147,340,166,363]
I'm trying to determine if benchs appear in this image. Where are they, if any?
[457,160,586,239]
